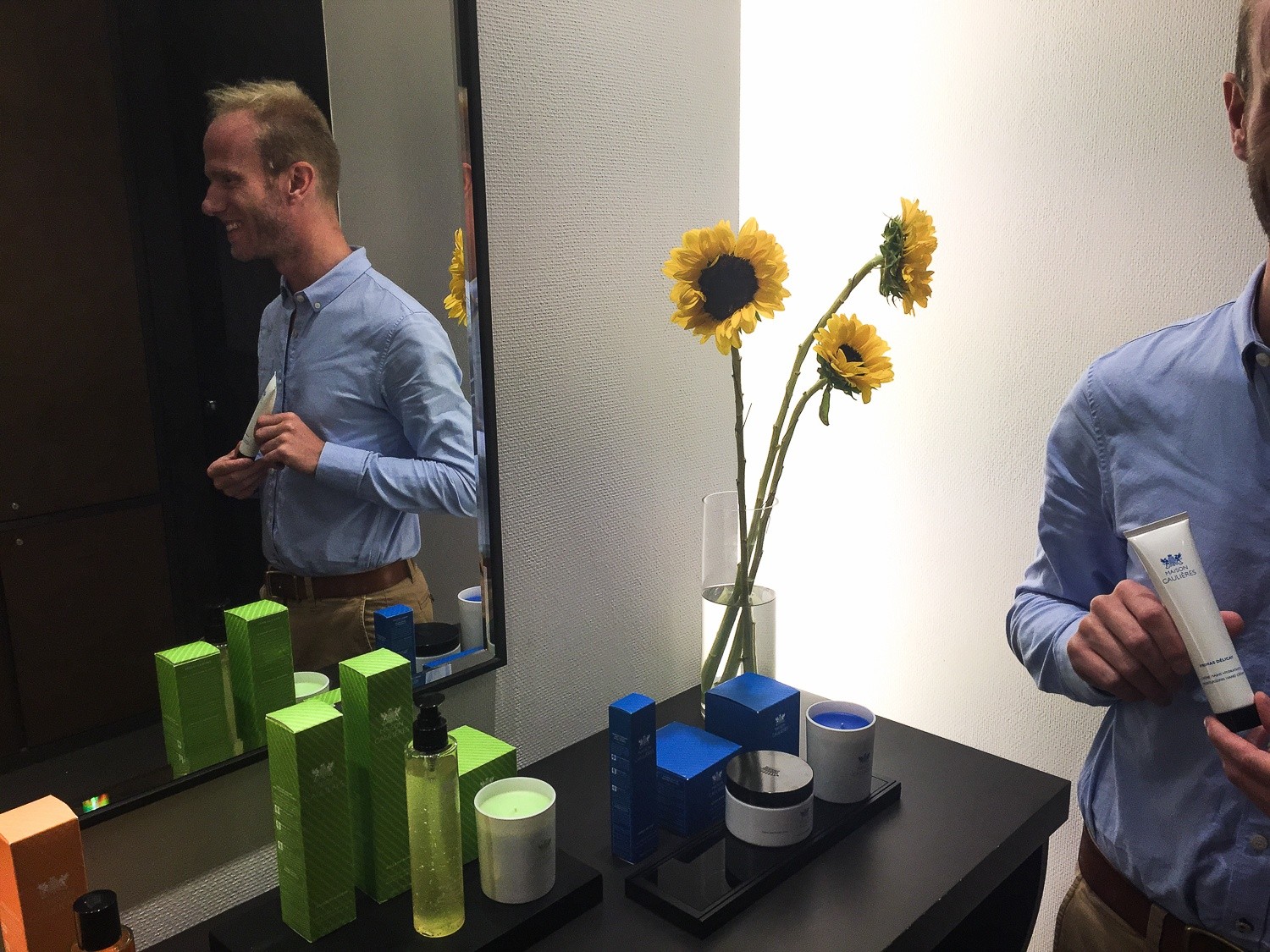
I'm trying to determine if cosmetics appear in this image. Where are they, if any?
[608,671,875,863]
[1121,511,1261,732]
[264,650,556,944]
[235,371,277,461]
[152,587,483,783]
[1,794,133,952]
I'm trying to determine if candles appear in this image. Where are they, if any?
[805,700,877,804]
[456,586,483,652]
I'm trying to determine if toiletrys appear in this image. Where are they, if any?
[413,622,461,674]
[238,370,277,461]
[212,642,244,756]
[724,749,815,849]
[70,887,138,952]
[1121,509,1265,735]
[403,690,466,941]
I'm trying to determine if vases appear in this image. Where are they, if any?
[698,490,782,723]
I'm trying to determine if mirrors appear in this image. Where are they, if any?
[0,0,509,832]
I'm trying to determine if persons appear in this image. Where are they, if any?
[1006,0,1270,952]
[202,83,475,697]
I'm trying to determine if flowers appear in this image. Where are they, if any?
[442,227,468,328]
[660,193,939,508]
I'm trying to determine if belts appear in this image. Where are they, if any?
[266,559,415,603]
[1079,828,1249,952]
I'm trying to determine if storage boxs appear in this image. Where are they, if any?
[373,604,417,675]
[608,693,659,866]
[656,719,744,839]
[704,671,801,757]
[223,600,296,740]
[0,795,89,952]
[154,640,232,764]
[265,699,356,943]
[339,647,412,904]
[452,724,517,866]
